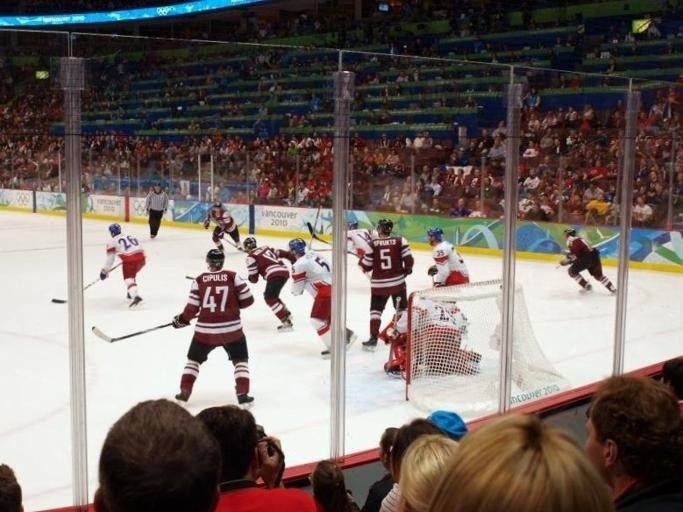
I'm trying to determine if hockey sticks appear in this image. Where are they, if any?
[186,274,250,281]
[92,322,174,343]
[52,262,123,303]
[307,222,359,258]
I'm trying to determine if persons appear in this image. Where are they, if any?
[1,360,682,511]
[0,0,683,233]
[172,181,471,409]
[100,224,147,307]
[559,227,618,295]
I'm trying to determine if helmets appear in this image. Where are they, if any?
[207,248,224,270]
[427,226,443,241]
[213,200,222,207]
[289,238,306,253]
[110,223,121,234]
[378,218,393,234]
[243,237,256,249]
[565,228,575,236]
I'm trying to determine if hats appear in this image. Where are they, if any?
[429,411,467,438]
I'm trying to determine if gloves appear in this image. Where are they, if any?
[173,314,189,328]
[100,269,107,280]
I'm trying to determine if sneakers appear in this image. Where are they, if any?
[277,312,293,329]
[322,350,331,355]
[579,284,592,294]
[238,394,254,403]
[129,296,141,307]
[611,289,617,294]
[363,338,377,346]
[175,393,188,401]
[346,328,353,344]
